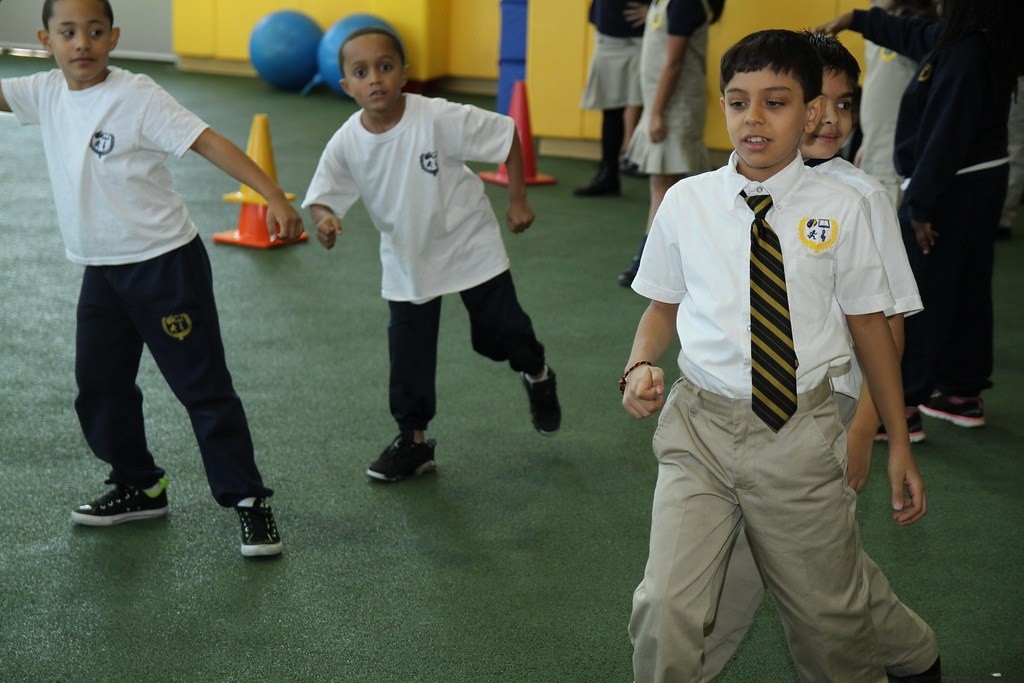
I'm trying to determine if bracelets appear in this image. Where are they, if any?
[619,361,654,396]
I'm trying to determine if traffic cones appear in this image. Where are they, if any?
[221,113,296,204]
[478,81,560,186]
[214,201,310,251]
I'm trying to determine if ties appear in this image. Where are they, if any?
[740,189,799,434]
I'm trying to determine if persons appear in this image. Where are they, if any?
[812,1,1023,442]
[572,0,726,287]
[620,28,940,683]
[0,0,304,557]
[301,29,561,483]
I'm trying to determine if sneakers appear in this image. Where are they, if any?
[234,496,283,556]
[873,409,926,442]
[367,429,436,482]
[918,393,986,429]
[70,475,169,526]
[522,365,562,436]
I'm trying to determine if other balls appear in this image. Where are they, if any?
[302,13,403,99]
[249,11,324,88]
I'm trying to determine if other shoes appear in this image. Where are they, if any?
[618,256,642,288]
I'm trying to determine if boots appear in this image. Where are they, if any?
[573,160,621,196]
